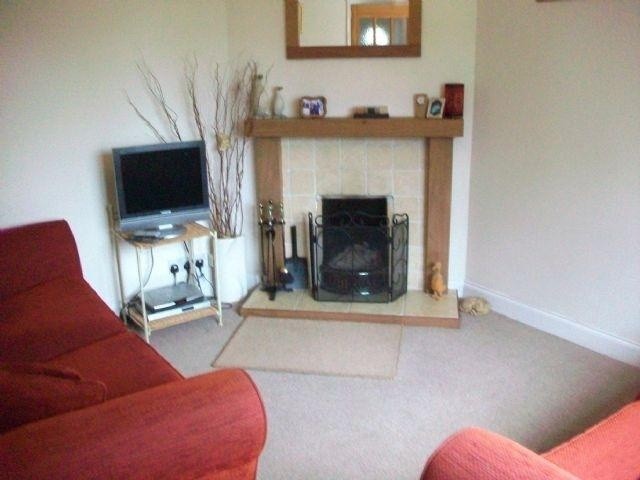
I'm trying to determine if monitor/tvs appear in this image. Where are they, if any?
[111,139,211,239]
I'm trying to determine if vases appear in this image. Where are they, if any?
[215,234,248,305]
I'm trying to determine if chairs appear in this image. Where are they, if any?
[420,391,638,480]
[2,217,268,479]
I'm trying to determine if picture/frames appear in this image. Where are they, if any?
[299,96,327,120]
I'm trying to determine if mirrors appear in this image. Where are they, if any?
[285,0,422,59]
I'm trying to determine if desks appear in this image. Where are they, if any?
[107,202,223,345]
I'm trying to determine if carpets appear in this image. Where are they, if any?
[210,316,404,381]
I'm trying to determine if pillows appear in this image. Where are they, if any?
[0,358,108,434]
